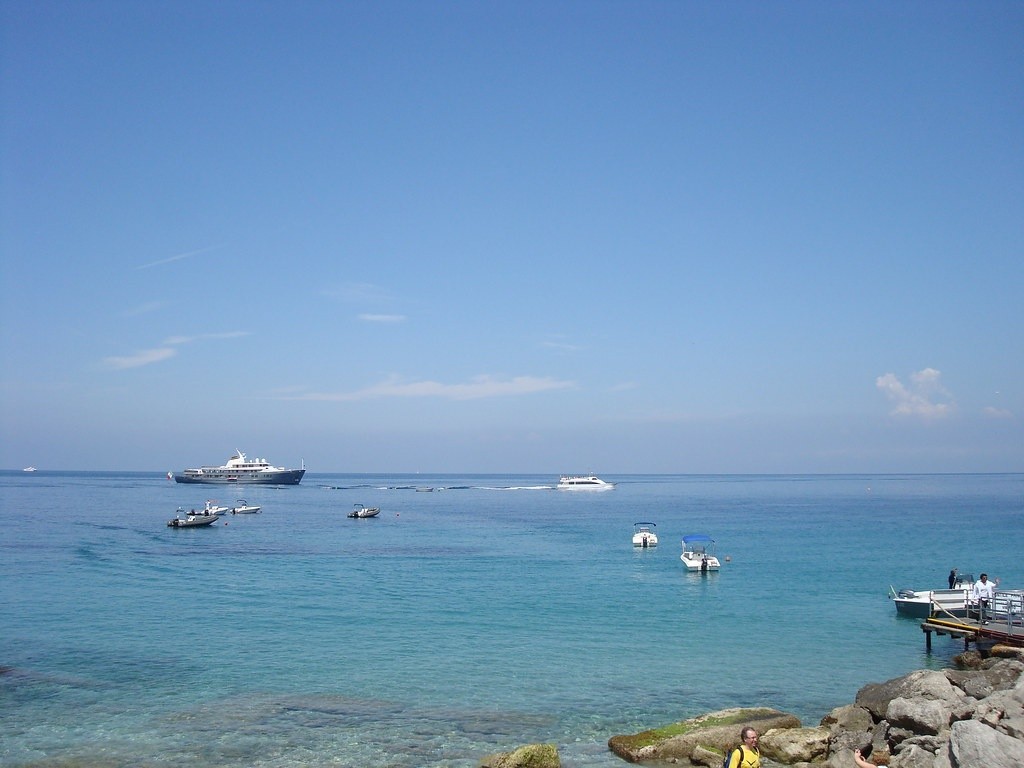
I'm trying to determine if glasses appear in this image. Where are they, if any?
[747,737,758,740]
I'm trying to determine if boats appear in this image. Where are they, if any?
[167,448,307,485]
[186,499,229,516]
[347,503,381,519]
[631,522,659,548]
[888,573,980,619]
[166,514,219,527]
[229,499,261,515]
[679,534,721,573]
[23,466,37,472]
[556,472,615,493]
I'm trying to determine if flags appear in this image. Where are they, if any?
[167,471,174,479]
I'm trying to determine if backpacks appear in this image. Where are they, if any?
[724,745,757,768]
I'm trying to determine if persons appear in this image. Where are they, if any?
[854,749,890,768]
[728,727,760,768]
[971,574,999,625]
[205,500,210,510]
[949,570,955,589]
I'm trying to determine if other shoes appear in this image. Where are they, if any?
[982,620,989,625]
